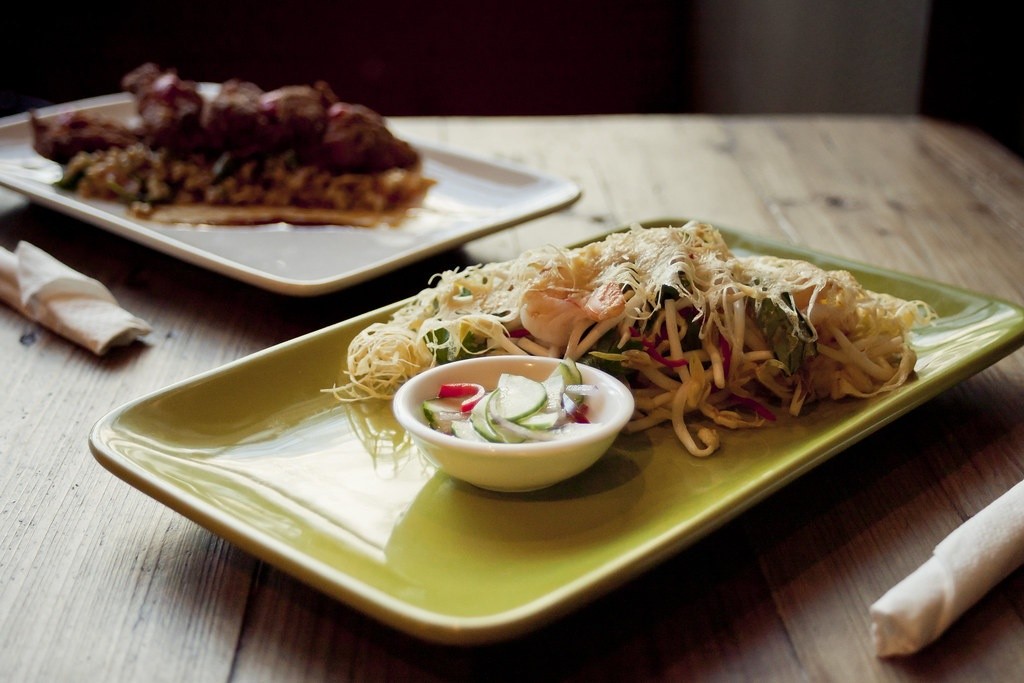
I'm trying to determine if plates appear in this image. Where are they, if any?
[85,218,1024,640]
[1,85,581,297]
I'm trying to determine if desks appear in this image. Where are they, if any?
[0,114,1024,683]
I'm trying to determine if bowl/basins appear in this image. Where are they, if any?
[391,355,636,493]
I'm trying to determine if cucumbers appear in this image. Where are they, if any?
[420,356,582,445]
